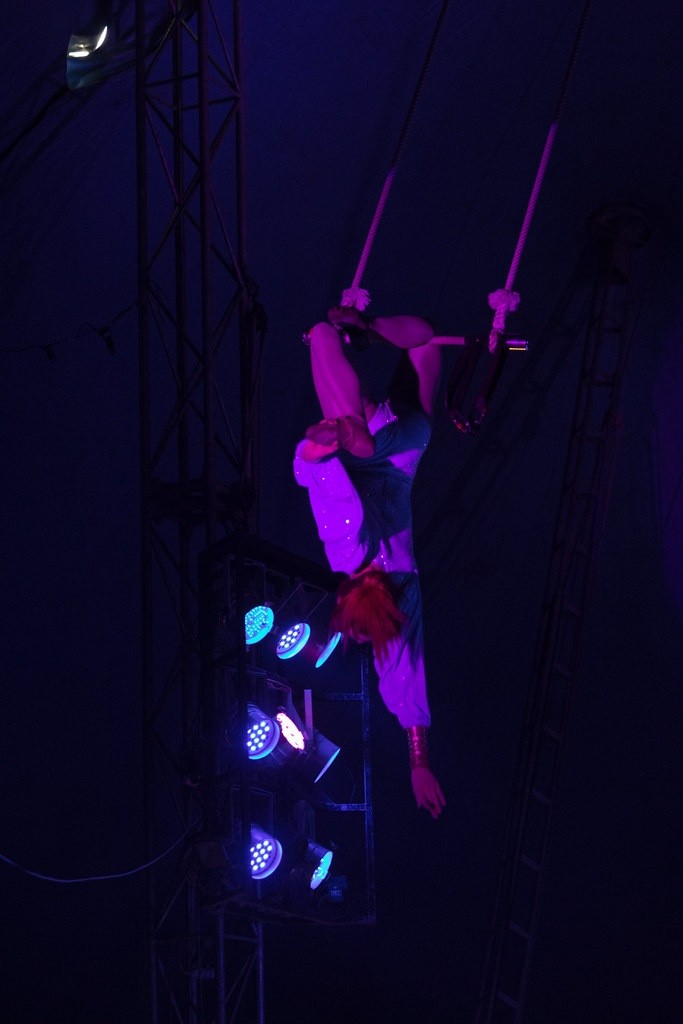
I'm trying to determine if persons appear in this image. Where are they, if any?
[291,315,452,816]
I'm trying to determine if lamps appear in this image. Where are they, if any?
[244,591,342,897]
